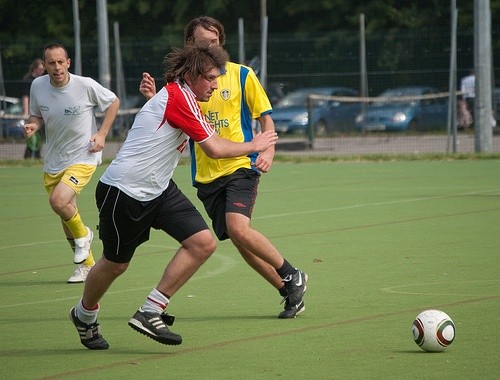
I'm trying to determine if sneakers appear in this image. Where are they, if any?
[280,267,309,308]
[72,225,94,263]
[277,295,306,319]
[66,260,96,284]
[69,305,110,351]
[128,306,182,346]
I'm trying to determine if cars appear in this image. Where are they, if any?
[356,84,448,136]
[265,87,362,136]
[0,96,26,135]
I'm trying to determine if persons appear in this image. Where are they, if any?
[461,74,477,126]
[140,17,309,319]
[21,60,44,159]
[70,38,279,350]
[23,43,120,283]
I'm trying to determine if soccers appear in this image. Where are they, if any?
[411,308,457,353]
